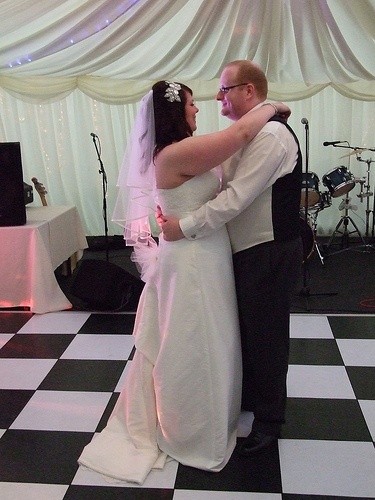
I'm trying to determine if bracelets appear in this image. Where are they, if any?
[262,103,278,115]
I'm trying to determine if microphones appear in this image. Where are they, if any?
[301,118,308,124]
[324,141,347,146]
[90,133,98,138]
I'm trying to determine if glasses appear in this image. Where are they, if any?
[220,83,248,95]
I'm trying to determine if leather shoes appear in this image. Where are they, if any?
[239,429,277,457]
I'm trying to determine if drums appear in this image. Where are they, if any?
[298,172,319,208]
[322,165,357,199]
[308,191,332,210]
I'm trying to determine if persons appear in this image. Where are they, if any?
[78,80,291,485]
[156,60,303,457]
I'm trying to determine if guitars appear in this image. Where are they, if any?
[31,174,48,207]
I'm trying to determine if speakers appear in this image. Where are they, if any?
[0,142,26,226]
[86,234,126,251]
[69,259,145,310]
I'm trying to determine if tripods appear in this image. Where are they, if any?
[289,124,375,297]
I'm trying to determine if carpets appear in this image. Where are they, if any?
[60,237,375,314]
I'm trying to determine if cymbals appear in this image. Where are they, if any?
[338,147,371,160]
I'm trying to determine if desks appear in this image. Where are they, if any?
[0,206,89,314]
